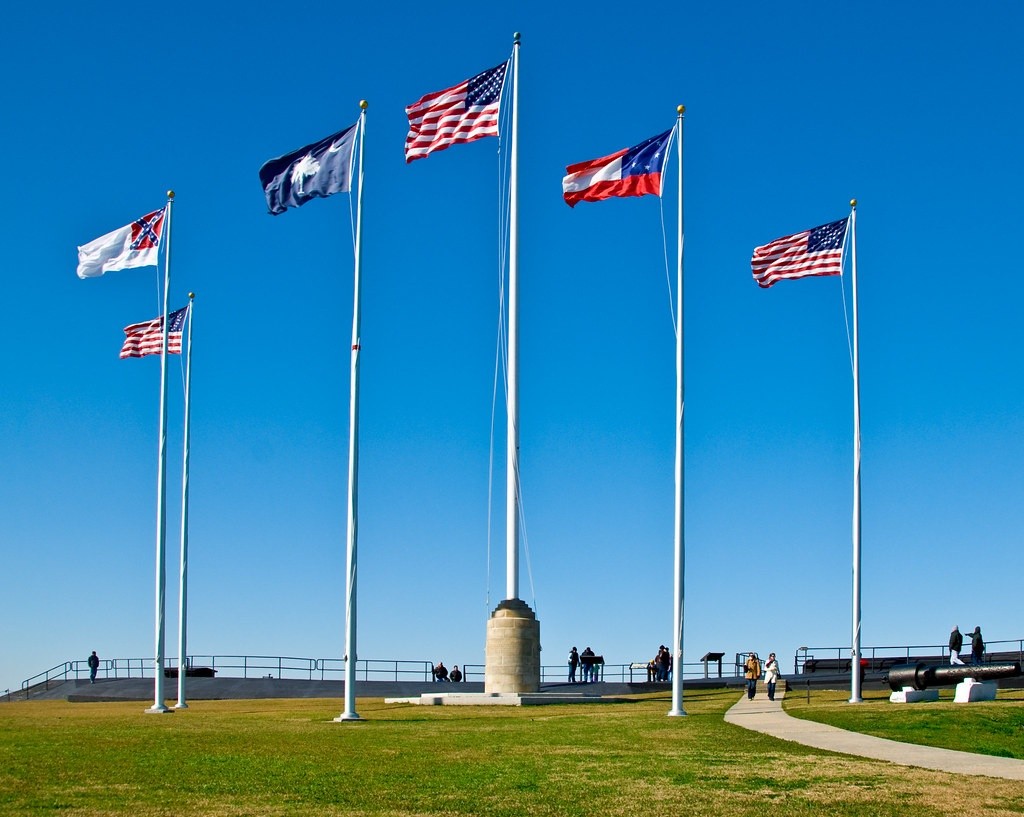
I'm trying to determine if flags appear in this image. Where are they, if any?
[75,205,168,279]
[404,58,510,164]
[751,217,851,288]
[561,125,675,208]
[258,117,360,216]
[118,303,189,359]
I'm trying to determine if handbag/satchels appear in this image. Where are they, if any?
[777,670,781,679]
[744,665,748,673]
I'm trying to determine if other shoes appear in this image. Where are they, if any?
[769,696,774,701]
[749,695,754,700]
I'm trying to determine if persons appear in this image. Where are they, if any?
[450,665,462,683]
[432,662,449,683]
[949,625,966,666]
[743,653,761,701]
[567,646,581,682]
[88,651,99,684]
[580,647,595,683]
[654,645,671,683]
[859,652,867,698]
[763,653,778,701]
[964,626,984,667]
